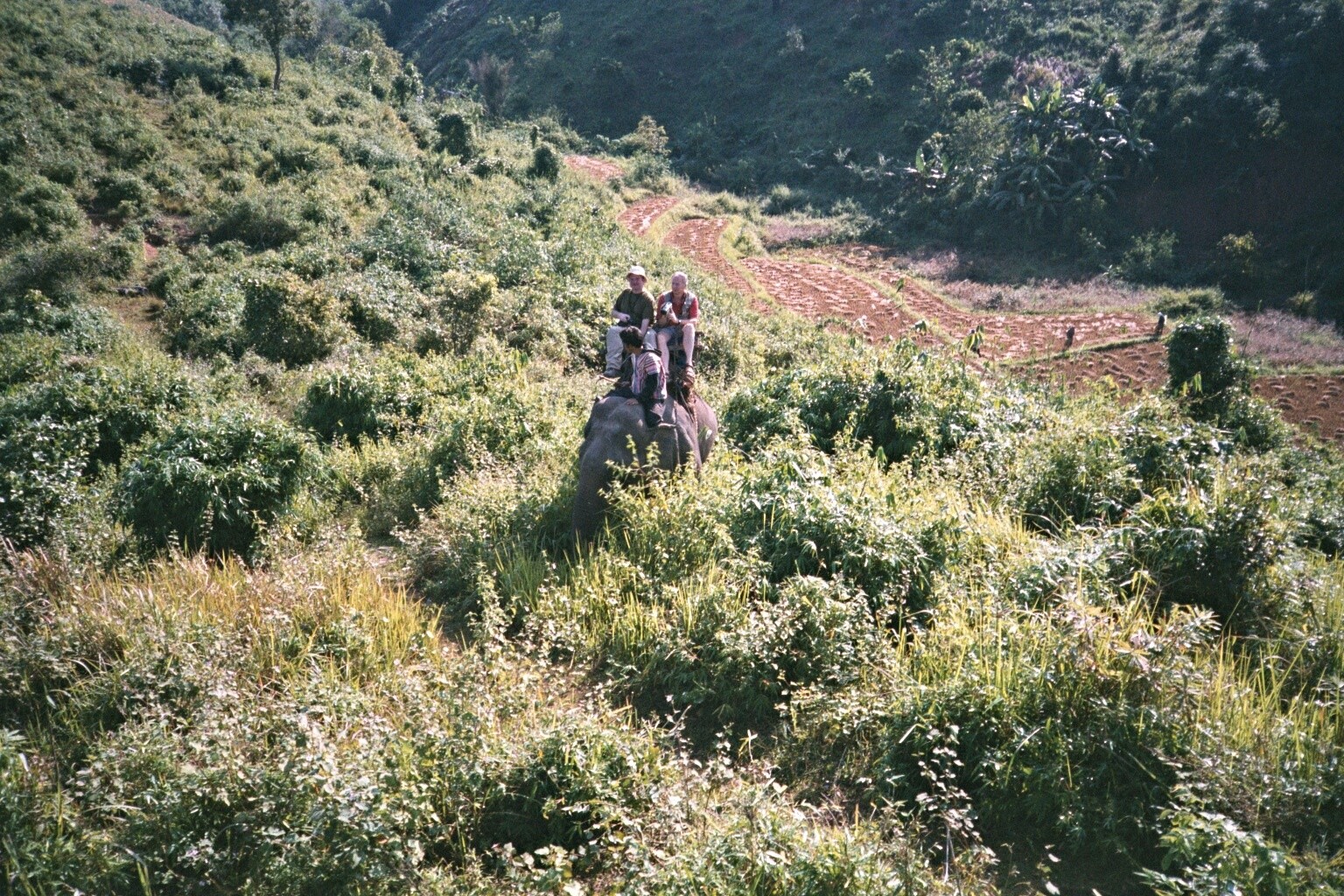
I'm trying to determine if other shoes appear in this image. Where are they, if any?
[603,368,620,376]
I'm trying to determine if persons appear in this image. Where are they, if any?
[967,328,981,357]
[596,326,668,427]
[656,272,699,377]
[1062,327,1074,352]
[1154,312,1165,335]
[604,266,656,377]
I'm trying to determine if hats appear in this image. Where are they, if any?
[626,265,647,282]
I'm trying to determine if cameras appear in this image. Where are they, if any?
[660,302,672,315]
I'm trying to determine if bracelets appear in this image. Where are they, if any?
[678,319,681,325]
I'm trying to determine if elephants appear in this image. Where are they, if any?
[574,395,718,541]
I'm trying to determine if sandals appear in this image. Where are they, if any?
[685,364,695,384]
